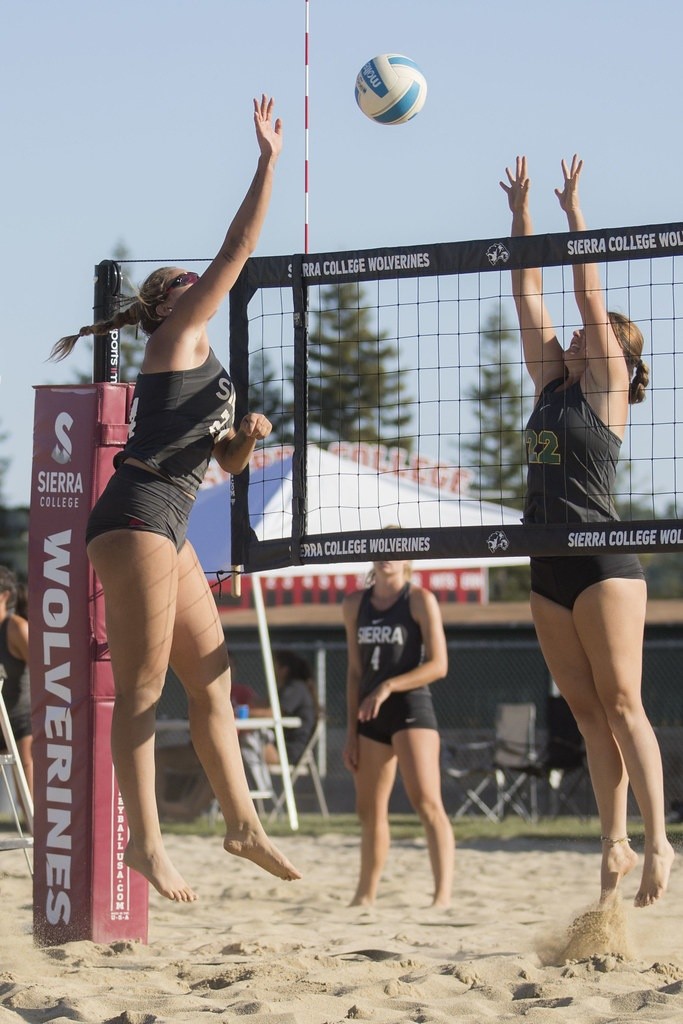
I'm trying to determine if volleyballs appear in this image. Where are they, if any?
[355,54,427,124]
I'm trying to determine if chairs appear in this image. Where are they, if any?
[445,698,541,827]
[260,711,323,832]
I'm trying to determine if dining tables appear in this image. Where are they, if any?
[152,715,317,819]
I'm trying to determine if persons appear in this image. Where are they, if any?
[500,153,676,909]
[157,650,318,824]
[224,649,259,716]
[0,562,33,836]
[47,92,302,903]
[341,525,456,910]
